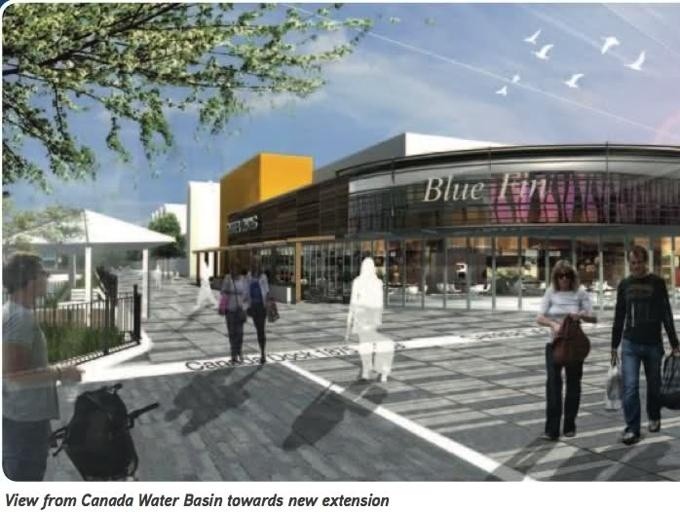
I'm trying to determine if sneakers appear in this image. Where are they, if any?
[646,418,663,432]
[618,429,641,444]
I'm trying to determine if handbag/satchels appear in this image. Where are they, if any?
[268,302,280,324]
[217,296,228,315]
[605,365,622,409]
[657,346,679,409]
[550,311,590,371]
[234,305,248,323]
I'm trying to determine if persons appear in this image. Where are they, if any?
[221,259,249,362]
[244,255,272,362]
[2,252,86,481]
[611,245,680,445]
[534,259,599,441]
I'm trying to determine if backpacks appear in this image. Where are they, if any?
[65,387,139,479]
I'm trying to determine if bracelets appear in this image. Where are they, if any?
[57,365,63,385]
